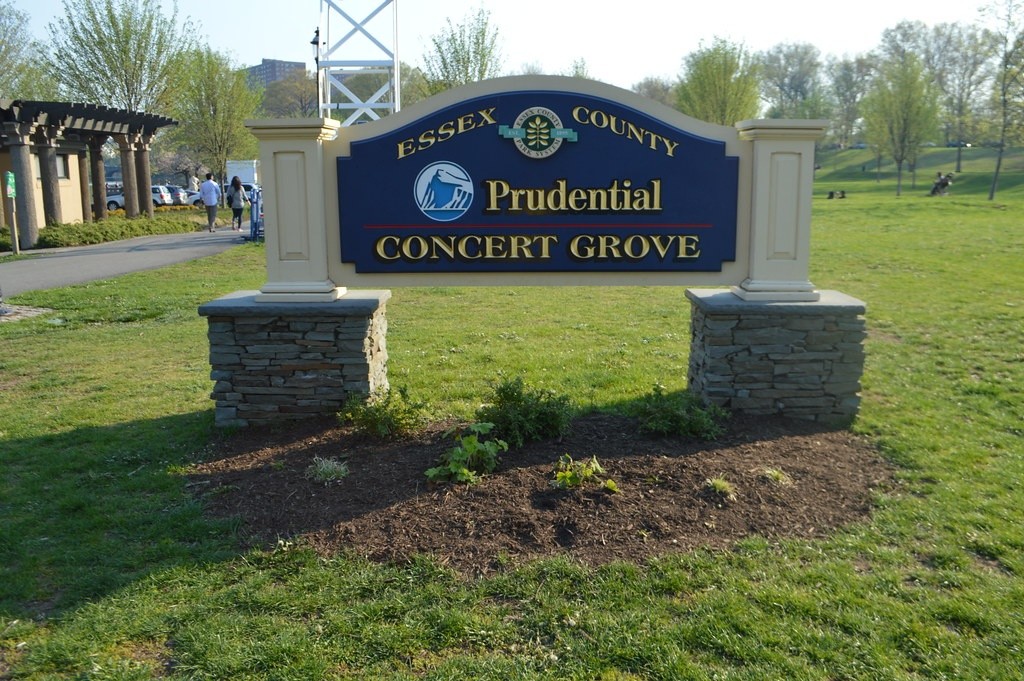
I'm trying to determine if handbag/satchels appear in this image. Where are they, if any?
[227,194,233,208]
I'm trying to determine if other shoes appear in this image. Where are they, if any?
[237,228,244,232]
[232,221,236,230]
[209,228,215,232]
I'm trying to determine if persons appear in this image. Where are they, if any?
[200,173,222,232]
[925,172,945,196]
[227,176,251,232]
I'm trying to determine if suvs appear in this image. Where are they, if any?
[107,183,173,211]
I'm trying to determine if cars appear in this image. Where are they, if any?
[188,183,260,206]
[848,144,865,149]
[185,189,200,196]
[946,141,971,147]
[918,141,936,147]
[165,185,188,205]
